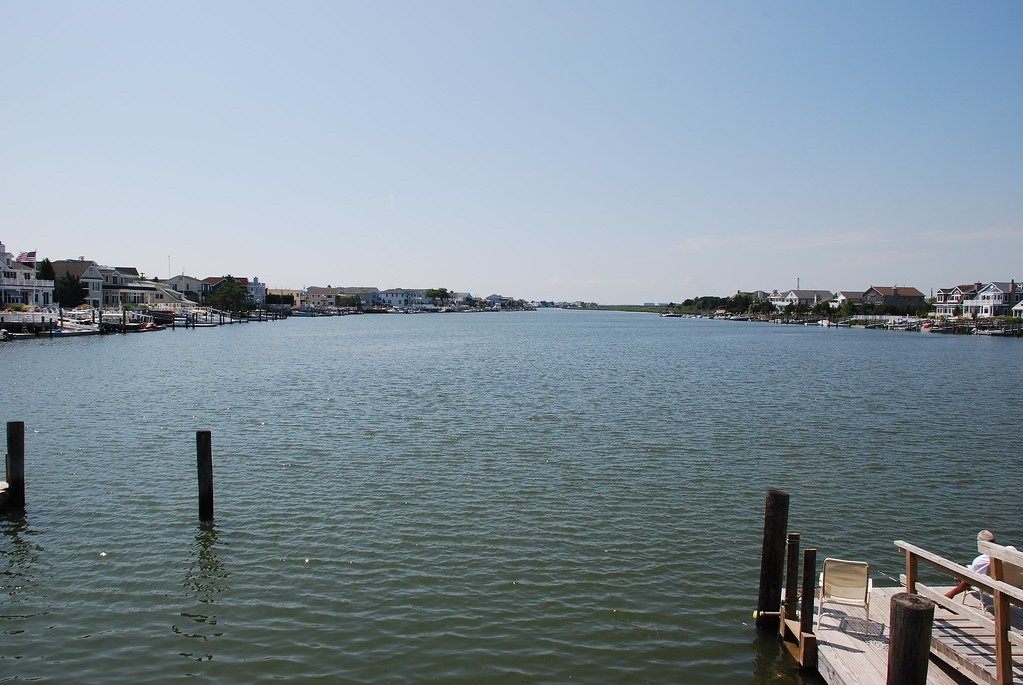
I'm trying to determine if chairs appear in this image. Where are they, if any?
[816,558,873,635]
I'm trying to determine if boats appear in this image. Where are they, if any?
[135,301,542,330]
[657,306,1015,342]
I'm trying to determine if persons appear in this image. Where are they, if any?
[3,305,52,313]
[944,530,1017,600]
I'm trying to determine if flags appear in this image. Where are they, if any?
[16,251,35,262]
